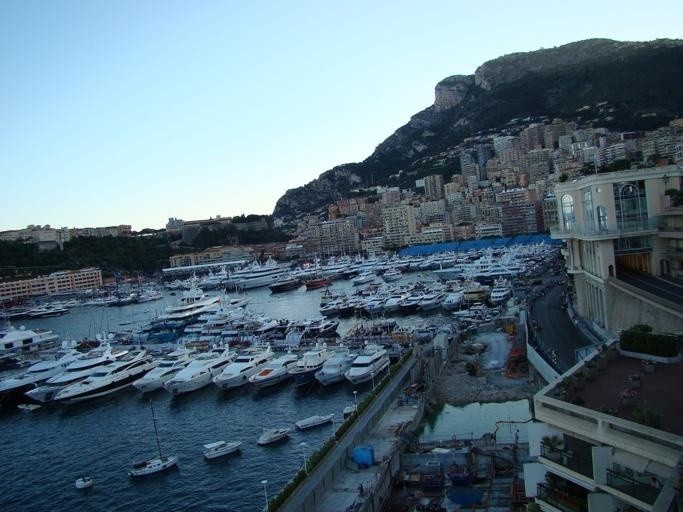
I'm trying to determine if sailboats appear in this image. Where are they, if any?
[127,399,178,479]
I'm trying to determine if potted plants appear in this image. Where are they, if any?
[550,350,664,428]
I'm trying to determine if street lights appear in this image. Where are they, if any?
[330,413,336,435]
[259,479,269,510]
[298,442,309,476]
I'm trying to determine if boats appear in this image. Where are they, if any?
[199,440,243,461]
[254,426,292,445]
[74,475,95,490]
[294,412,336,430]
